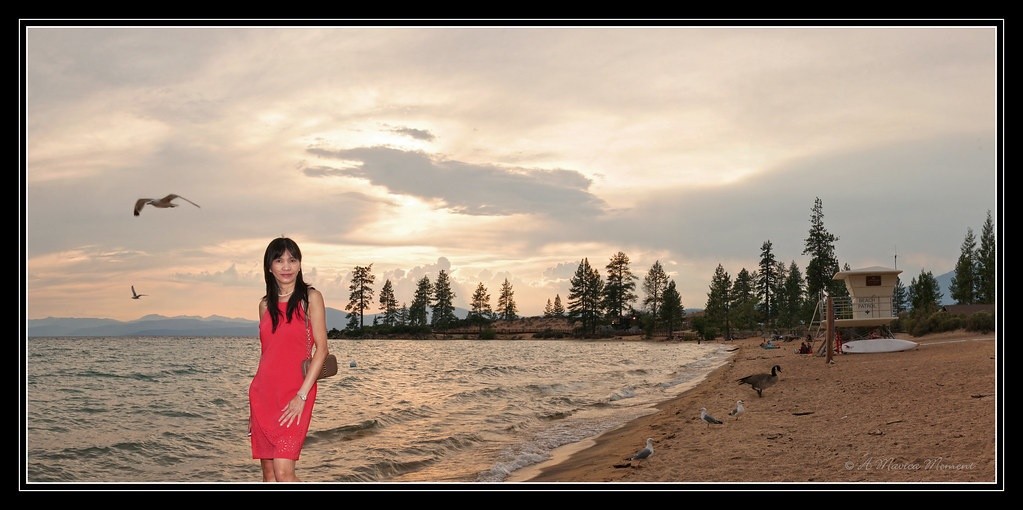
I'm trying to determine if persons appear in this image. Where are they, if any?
[248,234,327,482]
[794,342,812,354]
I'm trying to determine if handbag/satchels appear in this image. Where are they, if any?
[301,353,338,381]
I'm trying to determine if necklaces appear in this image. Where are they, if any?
[276,287,294,297]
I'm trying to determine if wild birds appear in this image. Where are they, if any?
[736,365,783,398]
[631,437,656,469]
[133,194,201,216]
[698,407,723,428]
[728,400,745,421]
[131,286,149,299]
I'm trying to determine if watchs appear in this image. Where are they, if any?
[297,392,307,400]
